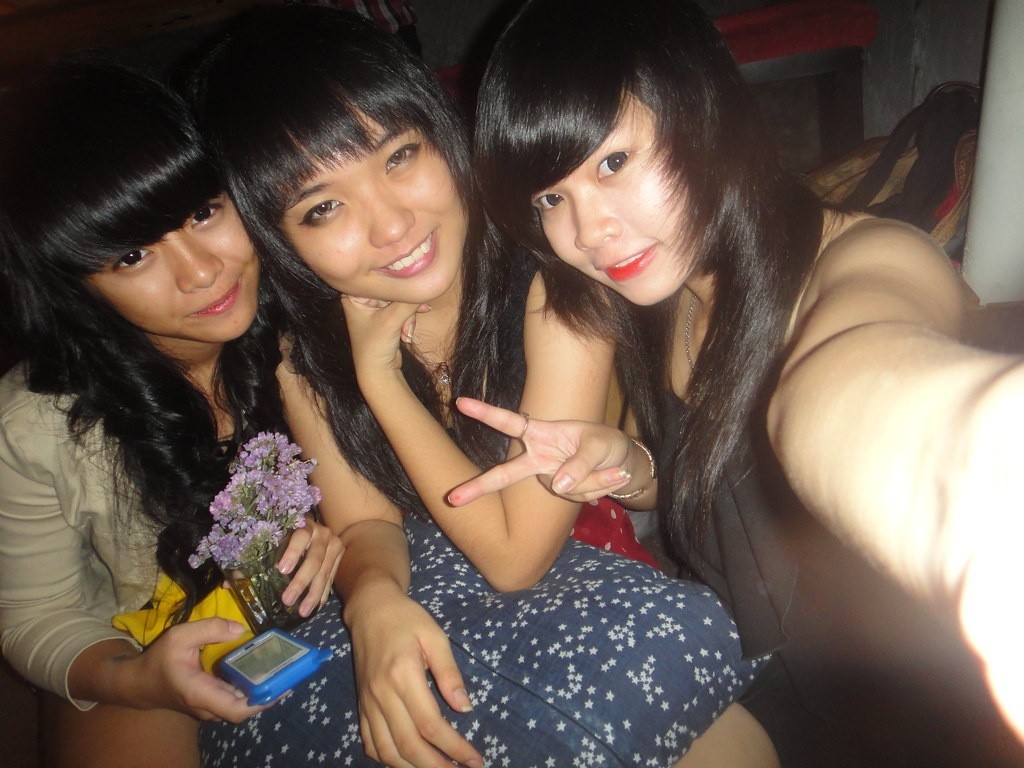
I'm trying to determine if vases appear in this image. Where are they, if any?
[216,533,319,633]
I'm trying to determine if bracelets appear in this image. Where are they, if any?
[606,437,655,499]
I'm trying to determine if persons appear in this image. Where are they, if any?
[190,0,779,767]
[0,39,346,767]
[445,1,1024,767]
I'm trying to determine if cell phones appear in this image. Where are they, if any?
[219,628,333,706]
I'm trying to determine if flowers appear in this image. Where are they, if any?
[187,431,324,615]
